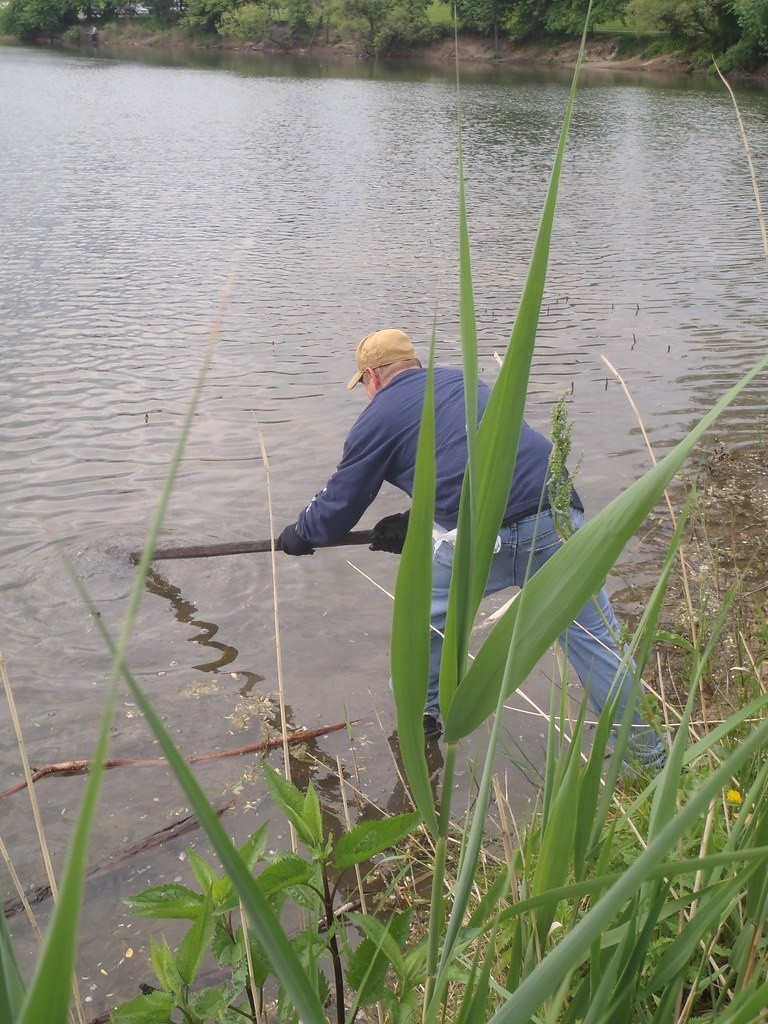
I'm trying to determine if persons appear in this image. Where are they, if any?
[277,328,689,777]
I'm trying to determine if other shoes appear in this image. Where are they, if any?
[424,714,441,740]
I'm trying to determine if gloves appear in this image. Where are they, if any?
[275,522,317,557]
[369,512,411,555]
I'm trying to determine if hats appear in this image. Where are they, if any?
[345,329,417,389]
